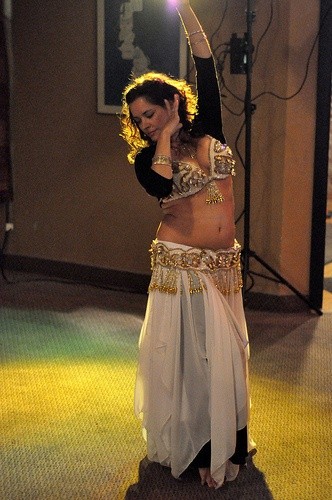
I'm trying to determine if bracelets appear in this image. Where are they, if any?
[189,30,206,44]
[152,155,173,166]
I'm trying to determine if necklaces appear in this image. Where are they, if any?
[182,145,196,159]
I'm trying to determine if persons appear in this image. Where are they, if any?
[122,0,250,487]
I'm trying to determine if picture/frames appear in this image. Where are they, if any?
[97,0,192,116]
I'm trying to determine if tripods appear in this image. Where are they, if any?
[236,0,323,315]
